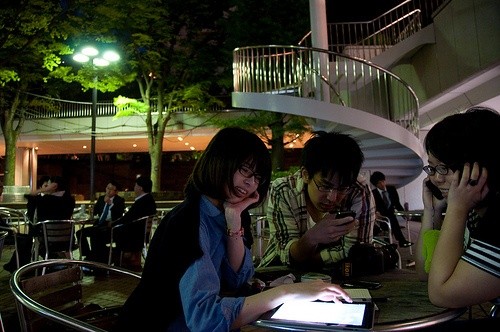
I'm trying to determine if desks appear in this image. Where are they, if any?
[394,210,423,255]
[76,218,98,227]
[248,266,468,332]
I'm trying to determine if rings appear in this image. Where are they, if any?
[469,179,478,187]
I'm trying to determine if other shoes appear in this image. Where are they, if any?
[399,241,413,248]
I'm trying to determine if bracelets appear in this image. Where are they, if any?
[227,227,244,239]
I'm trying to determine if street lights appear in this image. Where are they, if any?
[70,46,121,213]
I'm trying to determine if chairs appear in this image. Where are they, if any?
[252,215,270,261]
[0,207,158,332]
[373,213,405,270]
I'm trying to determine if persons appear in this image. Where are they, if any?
[412,106,500,332]
[371,172,414,248]
[257,130,375,270]
[76,182,125,261]
[0,182,32,274]
[111,125,354,332]
[93,175,157,265]
[23,175,76,260]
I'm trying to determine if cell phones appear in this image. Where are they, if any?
[336,210,356,225]
[425,179,444,201]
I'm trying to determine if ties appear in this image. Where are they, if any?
[382,192,389,206]
[99,203,110,221]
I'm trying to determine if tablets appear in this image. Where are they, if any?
[267,296,377,331]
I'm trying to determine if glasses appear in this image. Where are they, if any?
[423,162,458,176]
[312,175,353,195]
[239,164,266,186]
